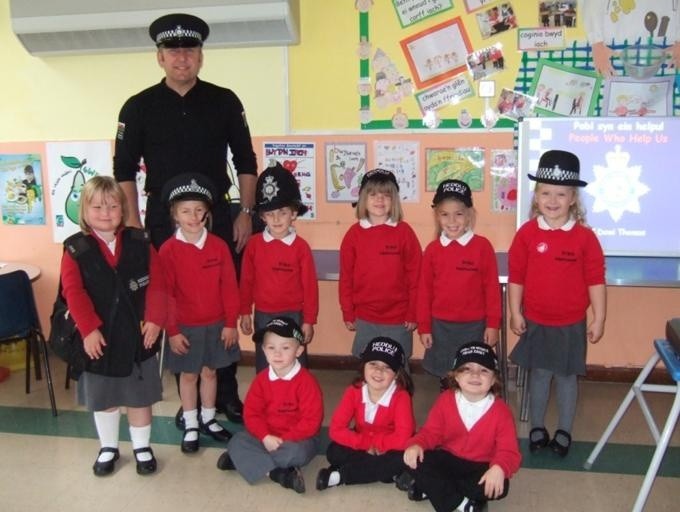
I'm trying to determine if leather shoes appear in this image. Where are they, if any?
[527,427,548,454]
[408,483,430,501]
[464,499,486,512]
[91,446,121,478]
[182,426,199,458]
[315,463,346,490]
[215,395,243,423]
[132,446,158,476]
[200,419,232,442]
[174,403,188,429]
[393,469,417,491]
[549,428,572,457]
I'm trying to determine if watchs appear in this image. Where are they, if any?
[239,205,258,220]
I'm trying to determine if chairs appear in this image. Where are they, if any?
[0,270,61,420]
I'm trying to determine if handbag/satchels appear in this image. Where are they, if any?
[47,231,139,381]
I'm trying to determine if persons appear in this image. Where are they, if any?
[59,176,166,476]
[507,150,608,457]
[215,315,324,493]
[316,335,418,493]
[399,340,522,512]
[112,12,260,430]
[235,160,319,376]
[157,170,242,456]
[337,168,422,380]
[413,179,502,389]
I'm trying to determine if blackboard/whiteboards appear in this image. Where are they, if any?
[517,115,680,260]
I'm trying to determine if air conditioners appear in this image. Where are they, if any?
[11,0,300,59]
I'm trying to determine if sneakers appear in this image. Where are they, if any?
[215,450,236,471]
[269,465,305,495]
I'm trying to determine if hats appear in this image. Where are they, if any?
[160,170,219,208]
[147,12,212,49]
[359,336,406,371]
[252,165,310,218]
[251,316,305,348]
[431,178,474,209]
[358,168,401,193]
[450,340,500,373]
[525,149,590,189]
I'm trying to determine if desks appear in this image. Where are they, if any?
[76,248,520,417]
[515,251,679,426]
[0,260,46,374]
[581,339,679,512]
